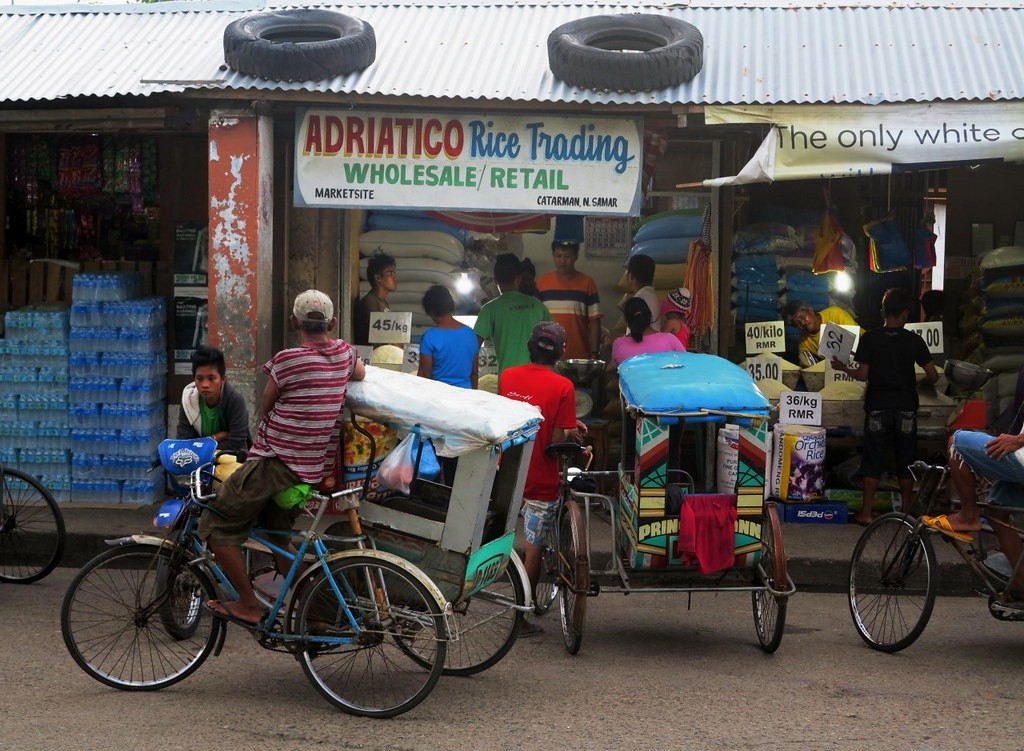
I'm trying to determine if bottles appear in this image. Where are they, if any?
[68,301,169,504]
[0,303,71,502]
[70,274,128,302]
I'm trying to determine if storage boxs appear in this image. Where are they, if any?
[784,500,848,524]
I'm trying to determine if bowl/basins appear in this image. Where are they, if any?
[915,371,944,386]
[801,370,825,391]
[782,369,800,391]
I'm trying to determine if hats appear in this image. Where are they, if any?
[661,288,691,320]
[293,289,333,323]
[532,320,566,351]
[622,254,655,283]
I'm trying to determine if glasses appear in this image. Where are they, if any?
[379,271,397,278]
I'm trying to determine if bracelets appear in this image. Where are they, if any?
[218,431,224,440]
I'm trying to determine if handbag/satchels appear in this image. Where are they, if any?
[376,430,413,495]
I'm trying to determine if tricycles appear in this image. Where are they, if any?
[0,466,66,584]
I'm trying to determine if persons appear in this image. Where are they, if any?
[200,290,364,662]
[787,288,1024,602]
[358,236,691,638]
[176,347,256,464]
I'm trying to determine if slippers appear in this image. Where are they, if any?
[517,624,544,637]
[848,514,870,527]
[306,627,329,659]
[202,599,260,631]
[922,514,974,542]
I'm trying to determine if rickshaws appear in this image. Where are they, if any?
[847,458,1023,656]
[531,351,796,655]
[60,364,535,718]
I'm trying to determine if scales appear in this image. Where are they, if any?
[564,359,606,421]
[943,359,993,431]
